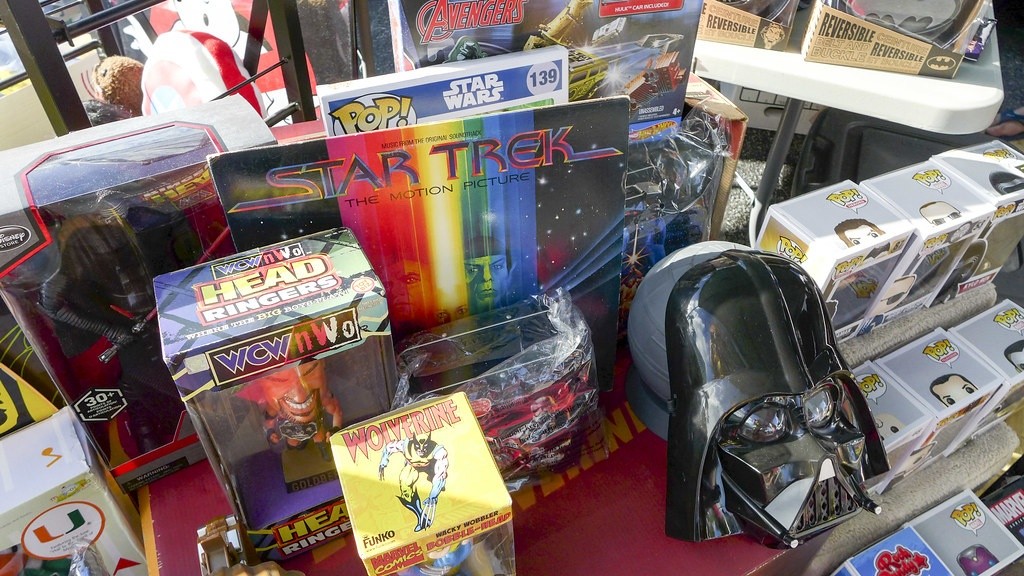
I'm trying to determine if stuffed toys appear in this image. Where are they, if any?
[82,56,145,127]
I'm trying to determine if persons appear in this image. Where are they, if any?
[37,180,217,455]
[235,357,344,463]
[984,106,1024,141]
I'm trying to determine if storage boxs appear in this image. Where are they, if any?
[695,0,985,79]
[0,93,515,576]
[754,139,1024,576]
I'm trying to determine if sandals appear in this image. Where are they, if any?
[985,110,1024,141]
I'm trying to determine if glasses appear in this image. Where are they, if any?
[735,389,836,443]
